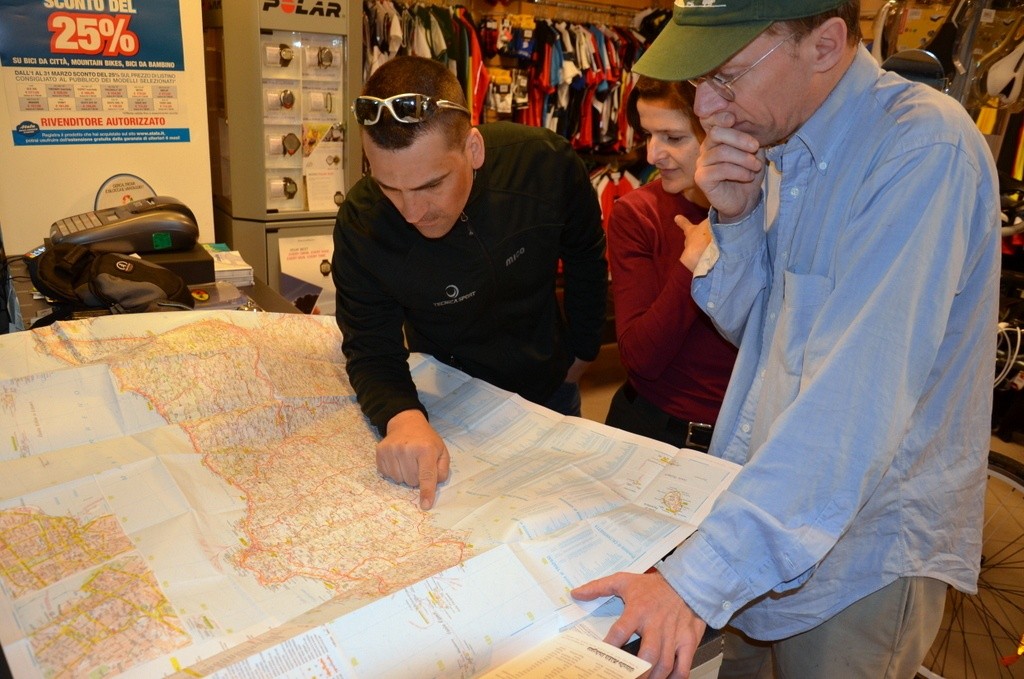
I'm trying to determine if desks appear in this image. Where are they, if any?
[0,253,728,679]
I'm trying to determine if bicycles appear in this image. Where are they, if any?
[908,448,1024,678]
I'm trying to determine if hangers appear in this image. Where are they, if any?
[374,0,639,33]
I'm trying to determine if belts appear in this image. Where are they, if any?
[620,377,715,453]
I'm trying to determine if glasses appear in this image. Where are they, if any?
[351,92,472,126]
[686,27,812,102]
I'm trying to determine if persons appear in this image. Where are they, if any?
[605,76,740,453]
[332,56,608,510]
[569,0,1002,679]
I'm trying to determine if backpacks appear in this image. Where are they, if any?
[20,236,196,332]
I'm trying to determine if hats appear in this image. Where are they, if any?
[630,0,861,82]
